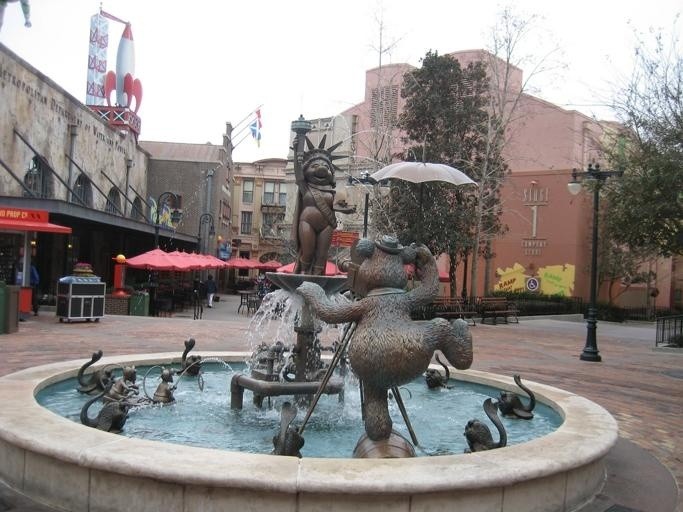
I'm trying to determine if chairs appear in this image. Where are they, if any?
[155,288,193,317]
[238,291,261,317]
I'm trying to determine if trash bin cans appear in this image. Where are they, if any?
[0,281,20,334]
[129,291,150,316]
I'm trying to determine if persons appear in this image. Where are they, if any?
[205,274,217,308]
[30,265,40,316]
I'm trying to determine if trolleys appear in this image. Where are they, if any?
[55,276,106,323]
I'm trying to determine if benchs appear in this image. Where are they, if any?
[479,297,519,324]
[431,298,477,326]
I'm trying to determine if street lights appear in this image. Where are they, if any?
[461,192,478,297]
[195,212,215,314]
[565,161,626,363]
[150,190,181,314]
[344,173,394,238]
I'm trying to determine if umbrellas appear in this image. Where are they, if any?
[369,135,479,246]
[404,264,450,283]
[124,245,348,275]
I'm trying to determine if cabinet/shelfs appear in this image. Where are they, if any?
[55,282,106,322]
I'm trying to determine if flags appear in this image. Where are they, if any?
[251,127,261,148]
[250,118,262,139]
[255,105,261,118]
